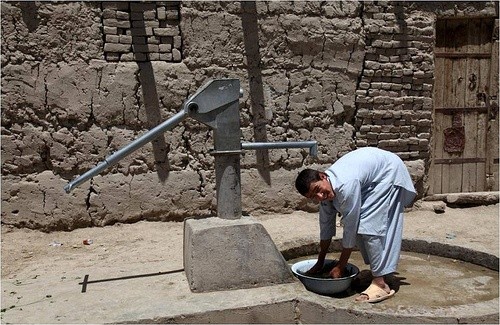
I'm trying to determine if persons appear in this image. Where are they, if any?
[295,146,417,302]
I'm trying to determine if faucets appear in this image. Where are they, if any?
[241,141,317,156]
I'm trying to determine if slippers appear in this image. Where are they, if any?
[354,284,395,303]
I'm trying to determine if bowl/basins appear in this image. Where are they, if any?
[291,258,360,294]
